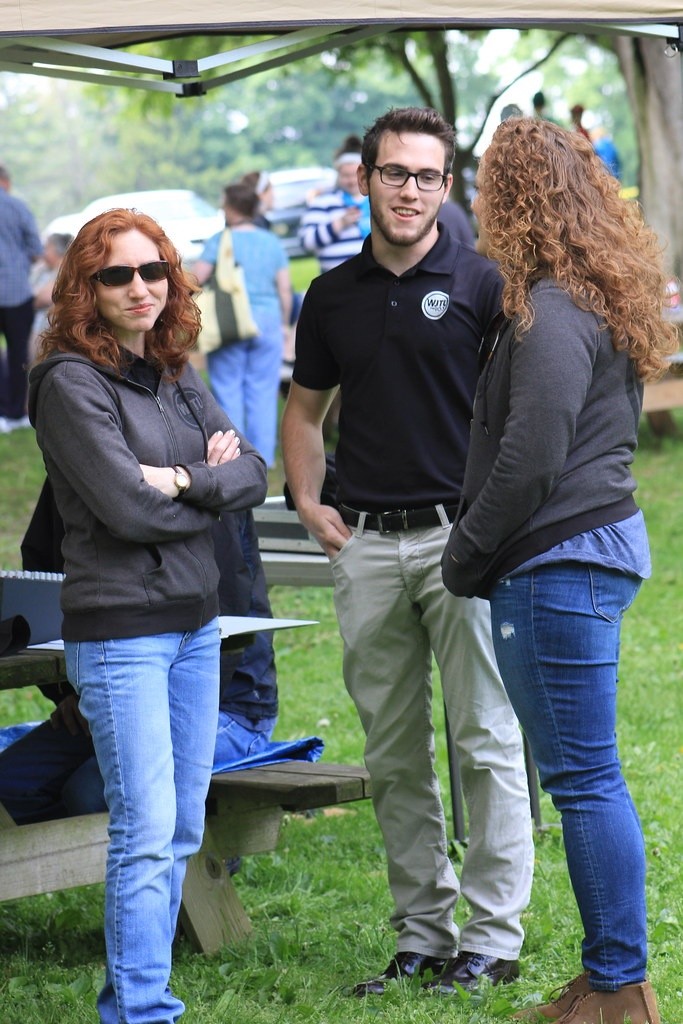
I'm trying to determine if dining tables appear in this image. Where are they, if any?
[0,566,259,962]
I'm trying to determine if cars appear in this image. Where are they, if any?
[270,166,337,213]
[42,189,233,263]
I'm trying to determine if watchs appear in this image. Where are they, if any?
[171,464,190,497]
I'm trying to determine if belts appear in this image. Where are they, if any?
[332,503,470,534]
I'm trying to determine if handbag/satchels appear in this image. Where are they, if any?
[187,229,260,356]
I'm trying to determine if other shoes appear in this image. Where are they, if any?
[509,971,661,1022]
[0,416,30,434]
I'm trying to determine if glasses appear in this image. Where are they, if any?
[88,260,171,287]
[368,162,447,193]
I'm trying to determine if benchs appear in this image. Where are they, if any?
[202,756,378,864]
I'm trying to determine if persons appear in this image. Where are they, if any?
[436,121,680,1023]
[23,229,74,427]
[25,208,268,1023]
[0,466,278,826]
[434,193,480,251]
[299,133,373,275]
[500,88,624,178]
[278,107,539,996]
[0,165,45,436]
[182,184,292,471]
[241,170,302,330]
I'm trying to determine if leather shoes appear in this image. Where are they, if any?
[347,951,459,997]
[419,947,521,998]
